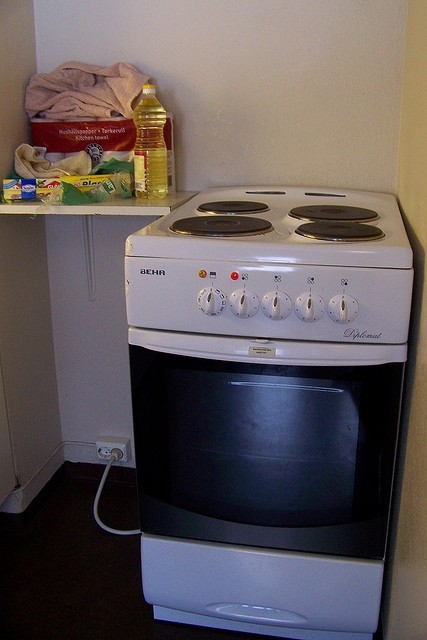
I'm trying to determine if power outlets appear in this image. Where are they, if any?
[96,436,132,462]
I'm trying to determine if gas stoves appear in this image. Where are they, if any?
[124,184,413,639]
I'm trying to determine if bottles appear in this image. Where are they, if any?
[133,85,169,200]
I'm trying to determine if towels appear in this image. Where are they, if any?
[25,62,151,117]
[14,144,91,177]
